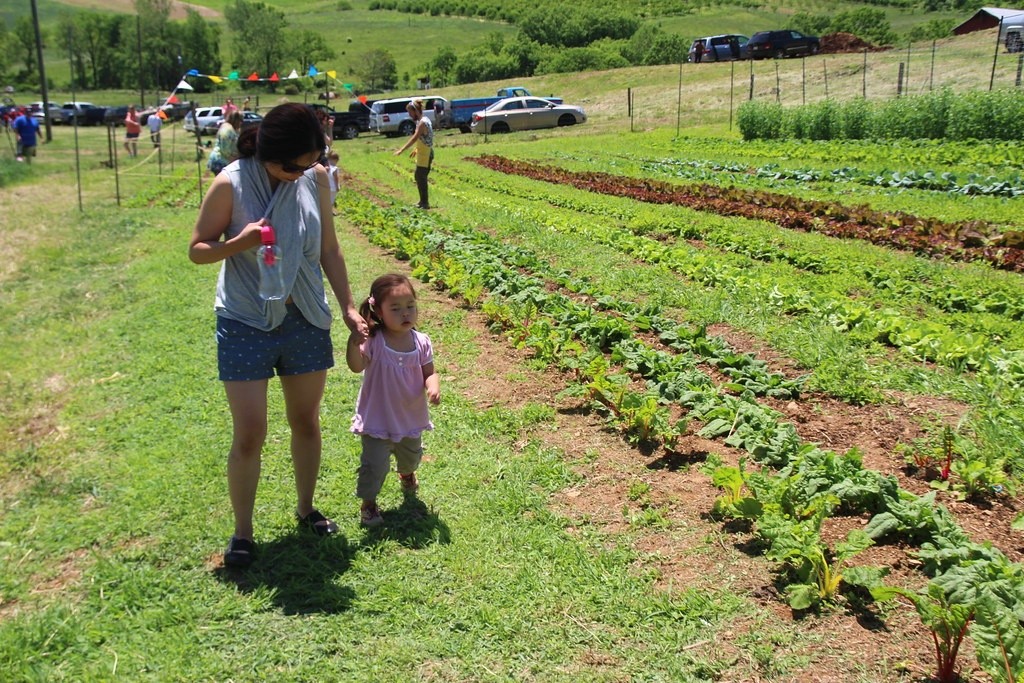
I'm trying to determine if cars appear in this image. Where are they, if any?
[470,96,588,135]
[30,101,148,126]
[184,106,263,137]
[687,34,750,62]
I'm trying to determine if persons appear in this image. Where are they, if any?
[345,274,440,526]
[188,102,367,566]
[147,114,162,147]
[5,105,43,164]
[207,96,251,176]
[124,103,141,156]
[728,38,741,60]
[395,100,434,209]
[694,40,705,63]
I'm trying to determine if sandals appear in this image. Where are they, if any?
[296,506,339,535]
[223,536,259,569]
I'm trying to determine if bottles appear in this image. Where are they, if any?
[256,224,285,300]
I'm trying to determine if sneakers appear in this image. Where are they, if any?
[403,498,426,510]
[361,505,385,528]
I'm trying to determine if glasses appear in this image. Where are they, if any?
[280,154,323,173]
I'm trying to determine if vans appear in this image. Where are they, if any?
[368,95,448,138]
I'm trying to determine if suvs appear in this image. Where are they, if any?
[746,29,821,60]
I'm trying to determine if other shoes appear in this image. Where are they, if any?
[416,203,430,211]
[128,151,137,159]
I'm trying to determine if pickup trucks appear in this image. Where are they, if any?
[433,87,563,133]
[307,100,377,139]
